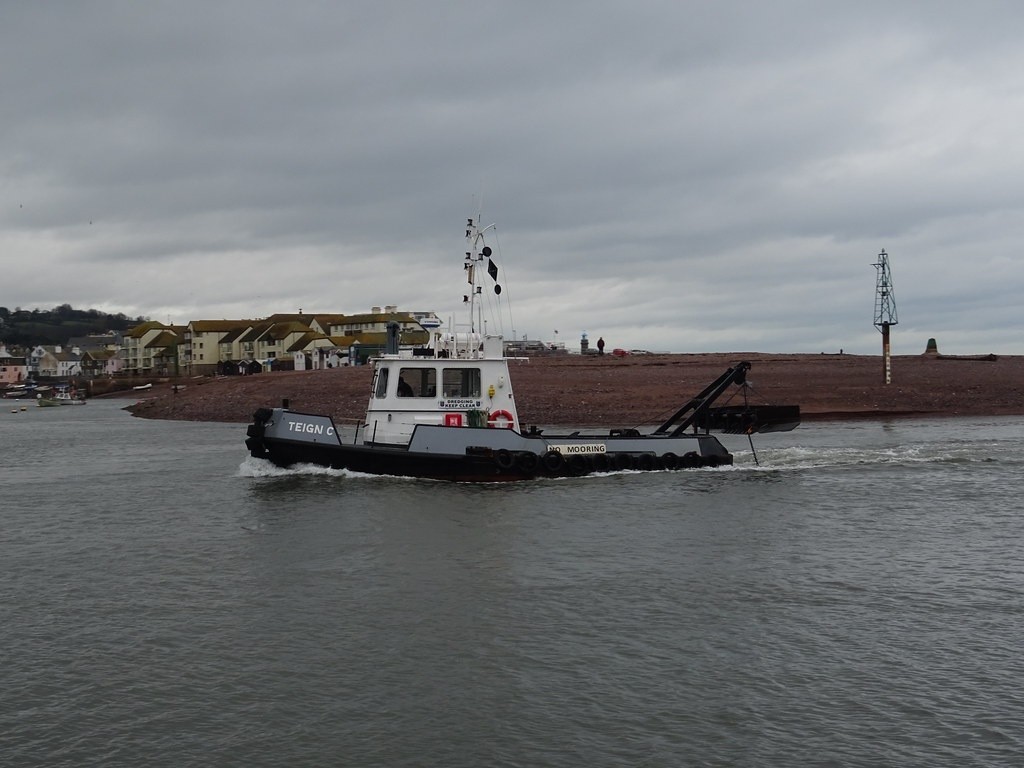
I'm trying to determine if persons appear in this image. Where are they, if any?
[398,377,415,397]
[597,337,604,355]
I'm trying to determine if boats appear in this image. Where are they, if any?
[133,383,152,390]
[13,383,25,388]
[35,385,53,391]
[5,390,32,397]
[38,390,87,407]
[25,386,37,390]
[247,332,750,481]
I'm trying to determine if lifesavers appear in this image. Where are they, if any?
[682,449,704,471]
[538,448,566,474]
[614,451,657,471]
[590,452,613,477]
[486,407,515,431]
[658,450,682,471]
[496,447,519,474]
[517,450,538,476]
